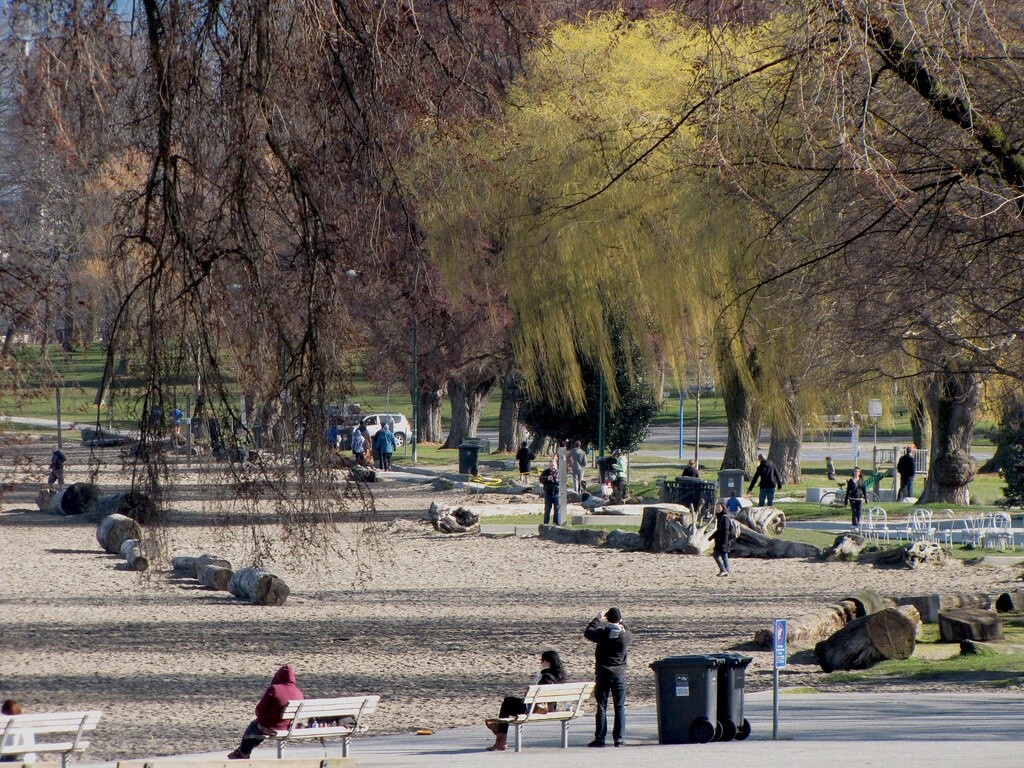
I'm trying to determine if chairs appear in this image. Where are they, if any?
[850,508,1015,553]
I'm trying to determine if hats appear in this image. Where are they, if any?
[51,445,58,452]
[759,454,765,457]
[384,424,389,430]
[604,607,620,621]
[367,419,371,421]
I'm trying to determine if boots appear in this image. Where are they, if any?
[485,718,507,750]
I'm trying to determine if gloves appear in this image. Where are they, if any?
[597,610,605,619]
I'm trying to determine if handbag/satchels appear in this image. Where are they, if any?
[525,702,548,714]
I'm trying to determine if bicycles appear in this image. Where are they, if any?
[820,482,881,515]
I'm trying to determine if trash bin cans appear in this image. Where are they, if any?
[191,417,218,448]
[675,476,703,513]
[457,444,483,476]
[717,469,745,498]
[709,653,754,742]
[648,655,726,745]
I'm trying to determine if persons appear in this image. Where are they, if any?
[516,441,531,482]
[486,650,568,750]
[153,402,184,435]
[46,444,67,484]
[708,502,735,576]
[724,490,743,515]
[747,454,782,507]
[559,439,629,504]
[538,460,561,525]
[845,466,868,526]
[682,458,699,478]
[897,447,916,502]
[329,420,397,471]
[825,456,836,480]
[228,665,304,759]
[584,607,634,748]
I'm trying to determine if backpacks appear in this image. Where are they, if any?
[722,514,741,543]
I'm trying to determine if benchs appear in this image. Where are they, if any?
[488,681,596,752]
[245,695,381,760]
[0,710,103,767]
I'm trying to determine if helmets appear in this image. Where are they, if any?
[613,449,621,454]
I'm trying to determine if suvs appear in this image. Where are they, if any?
[292,403,413,449]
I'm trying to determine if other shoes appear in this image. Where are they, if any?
[614,741,622,747]
[717,571,729,577]
[228,747,250,759]
[587,739,605,747]
[386,466,391,471]
